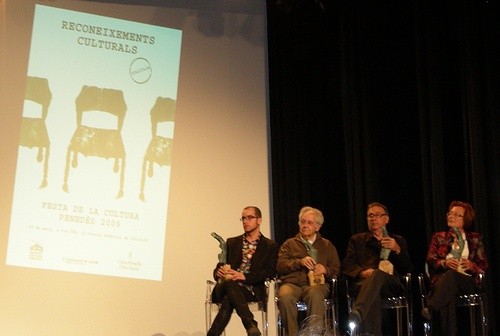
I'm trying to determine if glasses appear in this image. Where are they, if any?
[447,212,464,218]
[367,213,388,218]
[240,216,257,222]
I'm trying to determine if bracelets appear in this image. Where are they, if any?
[397,248,402,254]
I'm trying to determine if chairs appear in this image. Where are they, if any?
[204,278,268,336]
[344,272,414,336]
[276,276,338,335]
[418,270,489,336]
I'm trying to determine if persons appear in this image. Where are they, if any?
[341,202,412,336]
[206,207,278,336]
[422,202,490,335]
[277,206,342,336]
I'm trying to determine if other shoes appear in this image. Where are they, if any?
[247,325,261,336]
